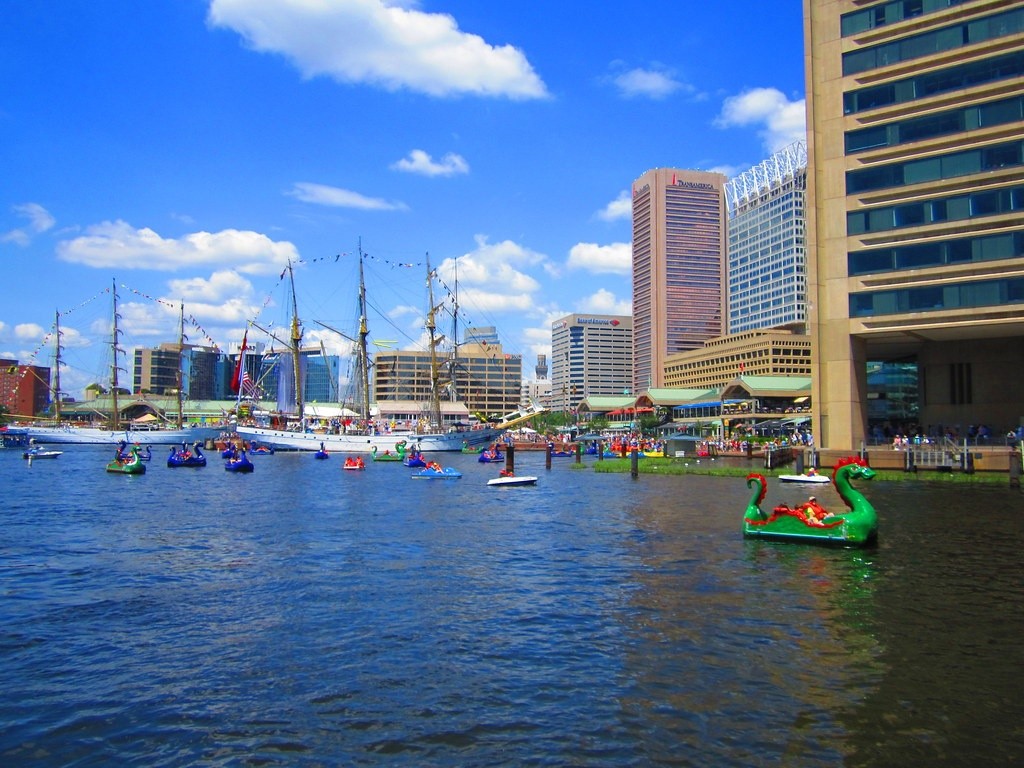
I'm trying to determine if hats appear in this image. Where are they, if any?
[809,468,814,471]
[434,463,437,465]
[808,496,816,501]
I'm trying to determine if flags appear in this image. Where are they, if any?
[231,338,260,404]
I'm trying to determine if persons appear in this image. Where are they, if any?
[499,469,514,478]
[871,424,990,444]
[914,434,920,445]
[702,436,743,452]
[893,434,902,449]
[788,430,812,447]
[1007,430,1017,450]
[803,496,835,522]
[901,435,909,447]
[502,430,568,443]
[922,434,929,443]
[429,461,444,473]
[484,449,498,460]
[622,434,661,450]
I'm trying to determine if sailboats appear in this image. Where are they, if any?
[0,277,236,445]
[237,236,551,452]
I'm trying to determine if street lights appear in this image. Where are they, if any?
[718,425,721,444]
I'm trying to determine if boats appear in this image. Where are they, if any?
[778,470,831,483]
[486,477,537,486]
[166,440,206,468]
[403,444,426,467]
[342,456,366,469]
[478,442,504,462]
[225,448,254,472]
[411,467,463,480]
[371,440,407,461]
[22,440,152,474]
[742,456,879,545]
[249,440,274,455]
[549,442,573,457]
[0,428,35,450]
[461,440,483,454]
[314,442,328,459]
